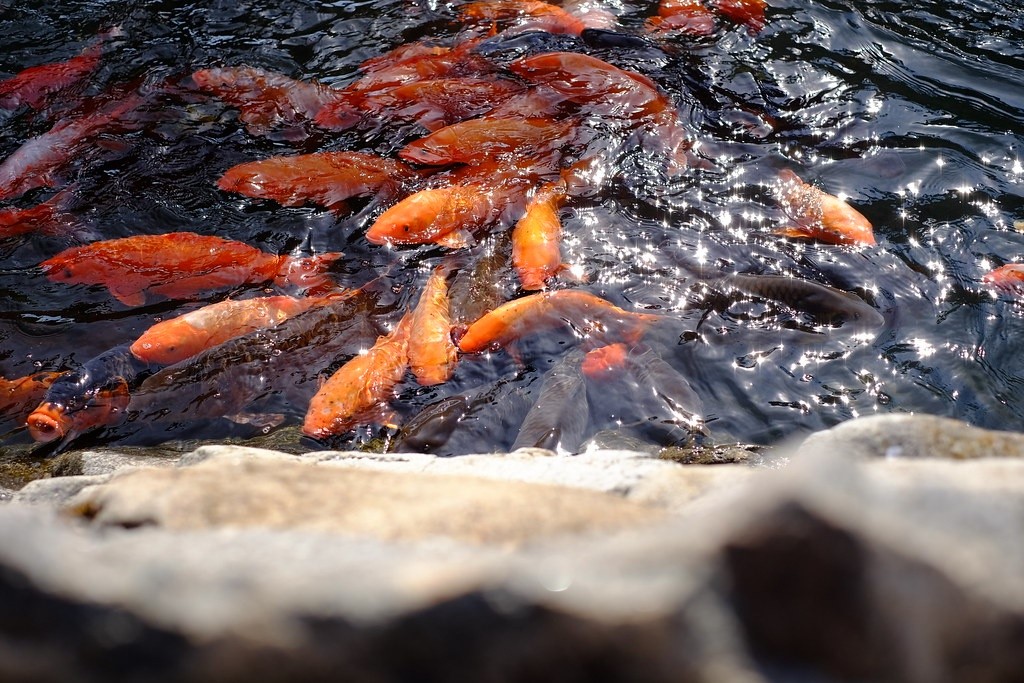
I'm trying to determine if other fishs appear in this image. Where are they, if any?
[0,0,883,455]
[982,262,1024,285]
